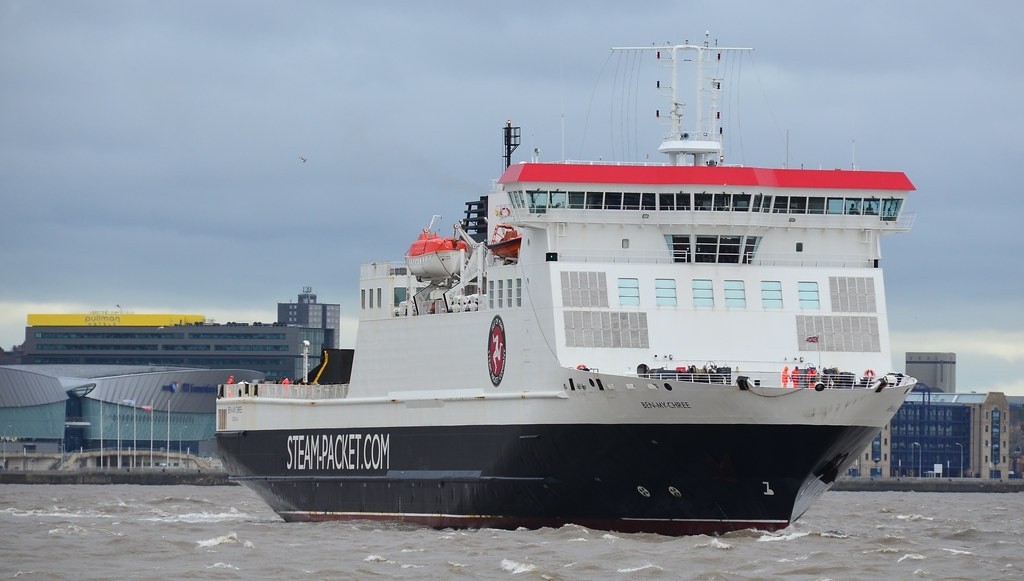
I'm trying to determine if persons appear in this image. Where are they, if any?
[790,365,800,388]
[782,366,788,388]
[806,367,818,388]
[227,376,235,398]
[282,377,290,398]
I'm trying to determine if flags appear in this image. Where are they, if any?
[122,399,135,406]
[807,337,819,342]
[142,405,152,411]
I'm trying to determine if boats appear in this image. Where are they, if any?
[485,232,522,260]
[402,225,471,282]
[214,29,922,537]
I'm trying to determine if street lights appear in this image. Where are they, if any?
[3,425,13,471]
[60,426,70,470]
[119,425,129,468]
[991,444,999,478]
[914,443,923,477]
[179,425,188,468]
[955,442,963,479]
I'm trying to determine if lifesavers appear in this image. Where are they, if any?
[577,365,587,369]
[500,208,510,216]
[864,370,875,377]
